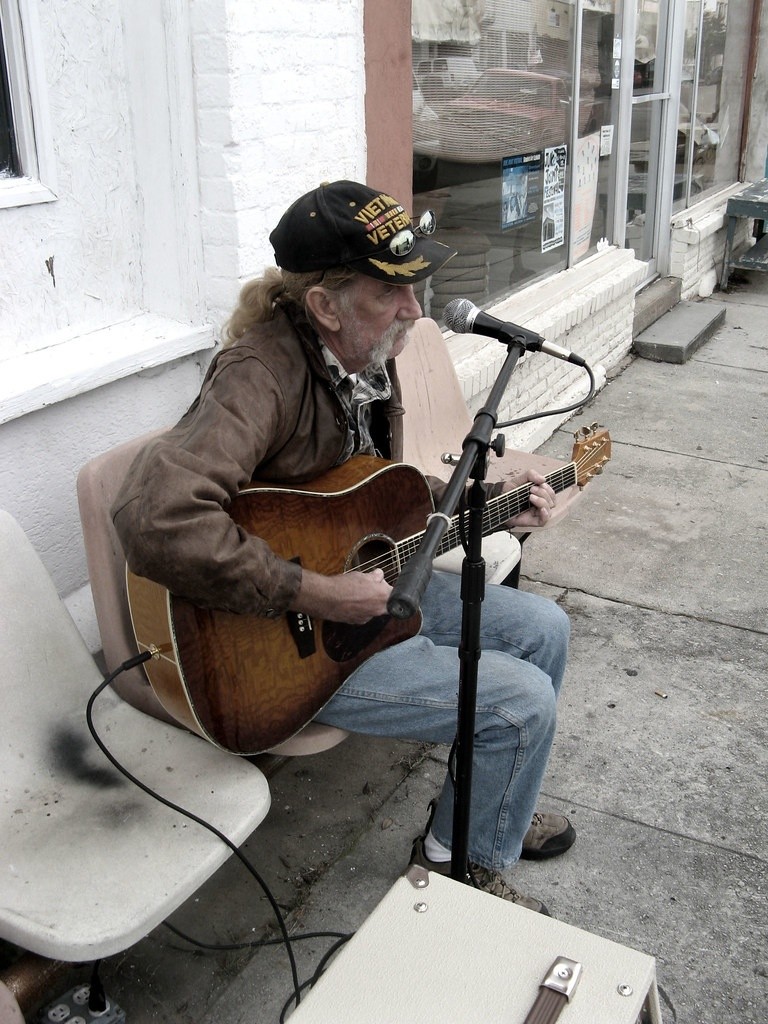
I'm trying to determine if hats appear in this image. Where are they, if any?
[268,180,458,285]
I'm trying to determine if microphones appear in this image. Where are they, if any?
[441,298,586,367]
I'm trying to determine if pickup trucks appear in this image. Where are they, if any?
[437,67,605,151]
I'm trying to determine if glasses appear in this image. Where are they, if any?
[316,207,436,284]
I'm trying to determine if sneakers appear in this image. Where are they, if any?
[424,793,577,862]
[404,835,550,918]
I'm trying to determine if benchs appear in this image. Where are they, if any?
[719,177,768,292]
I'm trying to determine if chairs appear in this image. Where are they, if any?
[395,317,592,533]
[433,531,522,590]
[0,510,283,1014]
[77,427,351,757]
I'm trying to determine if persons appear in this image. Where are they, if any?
[109,180,576,918]
